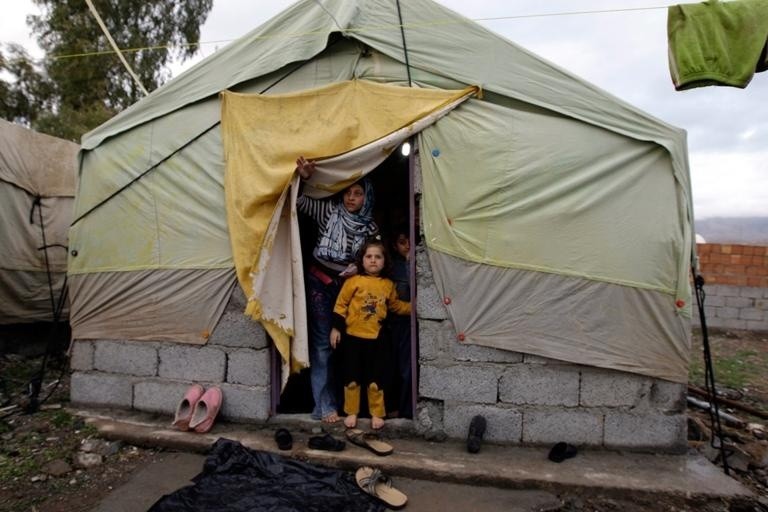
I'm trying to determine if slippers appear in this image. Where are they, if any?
[355,466,408,510]
[346,428,395,456]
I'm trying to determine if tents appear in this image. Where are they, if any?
[0,117,81,326]
[66,1,699,385]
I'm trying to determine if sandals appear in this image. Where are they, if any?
[276,428,292,450]
[308,434,345,451]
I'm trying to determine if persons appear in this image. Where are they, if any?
[296,156,382,423]
[330,231,413,429]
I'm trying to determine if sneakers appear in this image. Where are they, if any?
[467,415,486,453]
[175,384,223,433]
[549,442,578,462]
